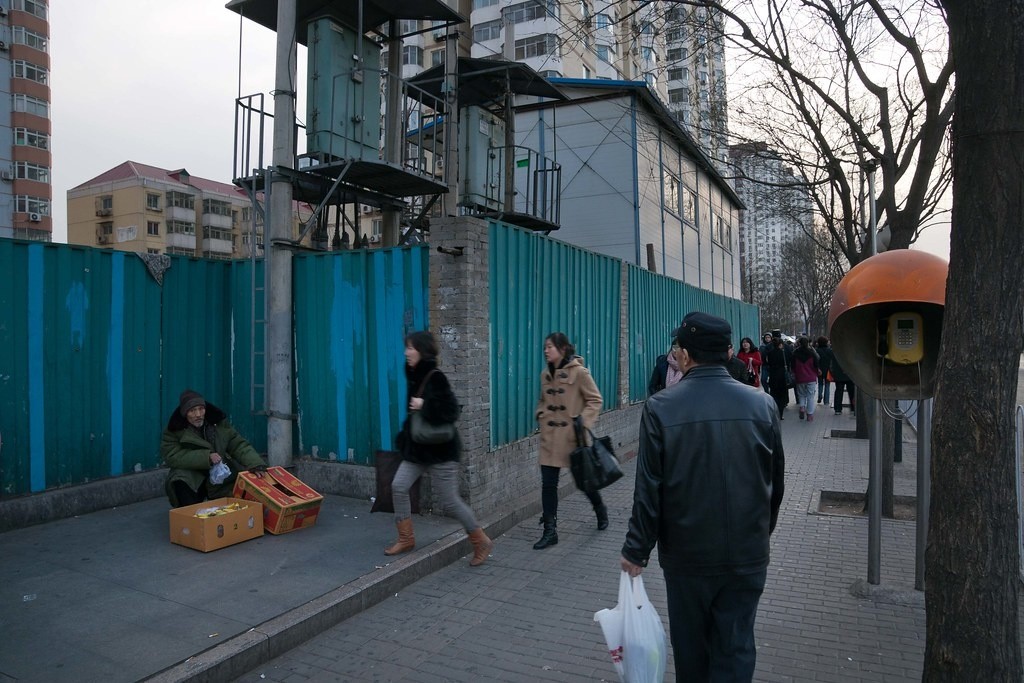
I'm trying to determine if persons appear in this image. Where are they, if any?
[383,331,493,566]
[725,338,762,388]
[621,312,785,683]
[648,338,684,396]
[759,329,793,421]
[533,333,609,550]
[160,390,268,508]
[792,332,855,424]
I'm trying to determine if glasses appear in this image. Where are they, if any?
[672,345,682,352]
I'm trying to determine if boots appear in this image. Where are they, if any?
[470,528,493,566]
[592,504,608,530]
[384,517,415,555]
[534,512,558,550]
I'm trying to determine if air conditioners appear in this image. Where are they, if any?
[0,5,8,16]
[0,41,9,50]
[370,235,379,242]
[433,32,443,41]
[362,204,373,213]
[98,235,106,243]
[29,212,41,221]
[436,160,444,168]
[2,170,14,180]
[95,210,101,215]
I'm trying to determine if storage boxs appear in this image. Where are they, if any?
[232,466,323,535]
[169,498,264,552]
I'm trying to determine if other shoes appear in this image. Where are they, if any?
[780,396,855,421]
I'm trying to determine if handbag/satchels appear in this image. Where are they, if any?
[785,372,795,389]
[209,459,231,485]
[746,358,756,383]
[410,369,455,444]
[569,416,623,492]
[370,451,419,513]
[593,569,668,683]
[827,369,834,382]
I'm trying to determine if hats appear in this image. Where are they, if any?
[671,312,731,352]
[180,390,206,417]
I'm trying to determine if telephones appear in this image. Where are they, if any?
[876,312,924,364]
